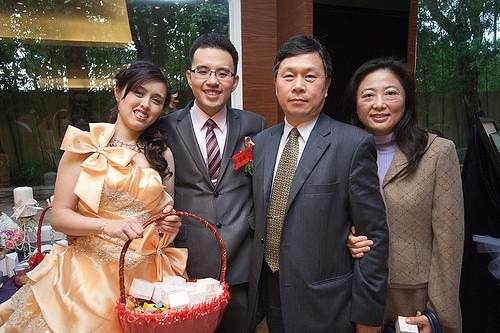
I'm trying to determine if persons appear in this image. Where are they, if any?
[250,35,389,333]
[0,61,192,333]
[9,101,94,186]
[346,57,465,333]
[152,33,270,333]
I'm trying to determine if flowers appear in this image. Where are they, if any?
[0,227,26,251]
[160,91,179,119]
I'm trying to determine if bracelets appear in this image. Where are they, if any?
[101,219,114,240]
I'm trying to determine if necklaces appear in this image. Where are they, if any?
[108,136,145,153]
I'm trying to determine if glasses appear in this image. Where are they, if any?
[190,65,235,79]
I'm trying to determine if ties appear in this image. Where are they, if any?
[260,128,302,272]
[205,118,222,185]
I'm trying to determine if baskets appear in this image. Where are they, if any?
[116,210,231,332]
